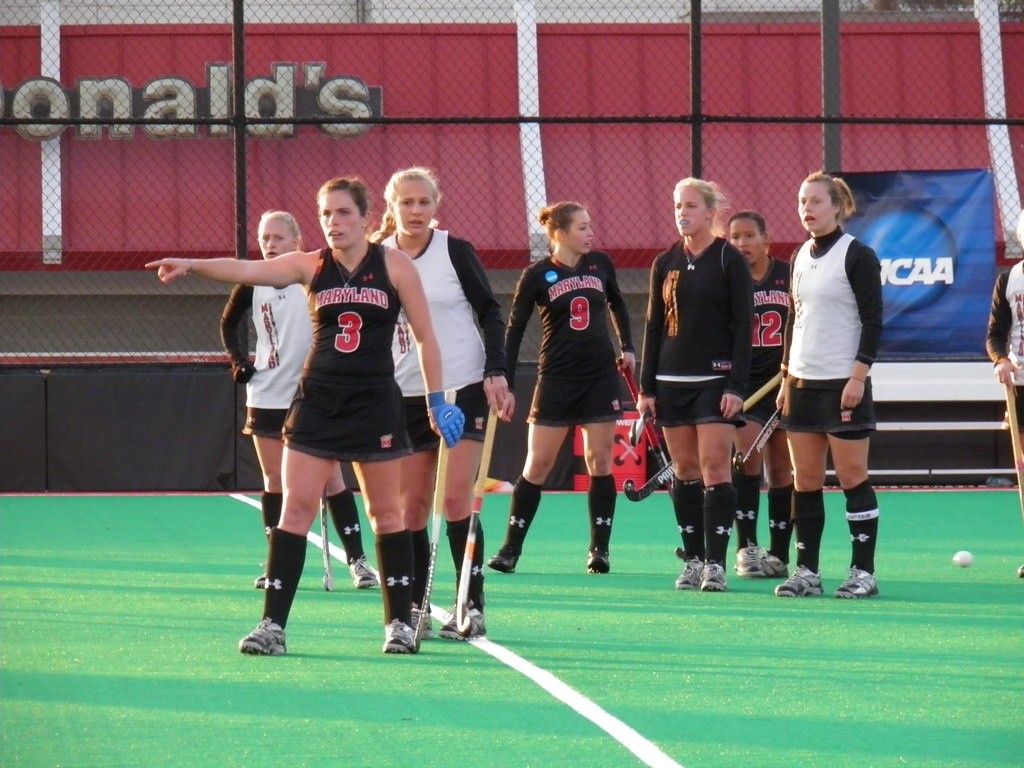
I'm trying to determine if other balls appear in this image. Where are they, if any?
[955,551,971,568]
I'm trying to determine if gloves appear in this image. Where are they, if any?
[426,390,466,446]
[233,364,258,385]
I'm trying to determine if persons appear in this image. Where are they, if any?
[728,211,791,578]
[776,172,883,600]
[487,202,634,573]
[635,178,754,591]
[145,178,464,653]
[220,211,379,588]
[985,210,1023,578]
[370,168,509,639]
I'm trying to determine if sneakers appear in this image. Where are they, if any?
[437,597,487,642]
[382,618,420,653]
[587,548,611,574]
[348,556,379,589]
[700,563,728,591]
[775,565,825,598]
[759,553,789,579]
[486,545,520,573]
[833,567,879,599]
[238,617,288,656]
[409,608,435,641]
[675,556,706,590]
[733,547,765,578]
[254,571,267,589]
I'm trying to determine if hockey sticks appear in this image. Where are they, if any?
[733,404,783,476]
[319,486,333,592]
[623,370,782,503]
[629,407,650,447]
[618,356,673,495]
[413,387,457,653]
[1004,382,1024,579]
[455,405,499,637]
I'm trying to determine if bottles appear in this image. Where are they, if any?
[987,477,1014,487]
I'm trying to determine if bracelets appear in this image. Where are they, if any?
[852,377,864,383]
[781,369,787,378]
